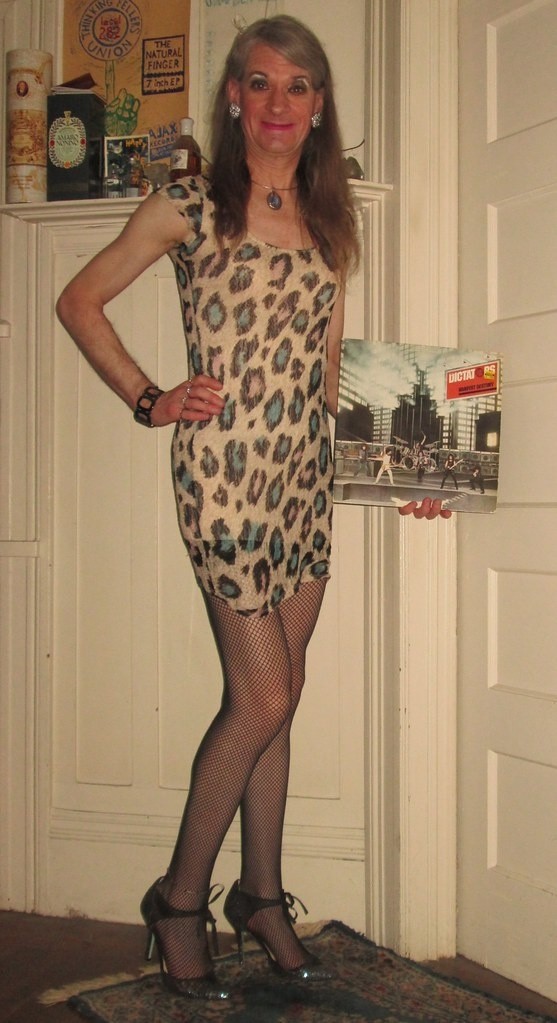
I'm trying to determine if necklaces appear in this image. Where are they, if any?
[251,180,298,211]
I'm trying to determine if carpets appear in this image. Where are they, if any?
[37,919,556,1023]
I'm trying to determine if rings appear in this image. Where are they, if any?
[188,375,197,385]
[182,397,190,409]
[178,409,185,420]
[186,386,192,398]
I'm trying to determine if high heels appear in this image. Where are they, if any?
[140,876,232,1000]
[223,879,338,982]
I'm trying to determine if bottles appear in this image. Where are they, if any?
[170,117,202,180]
[104,163,123,197]
[123,157,140,197]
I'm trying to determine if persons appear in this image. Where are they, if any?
[354,436,485,494]
[56,13,452,999]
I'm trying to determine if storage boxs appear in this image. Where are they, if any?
[44,93,107,202]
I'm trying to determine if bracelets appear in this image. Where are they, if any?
[133,386,165,428]
